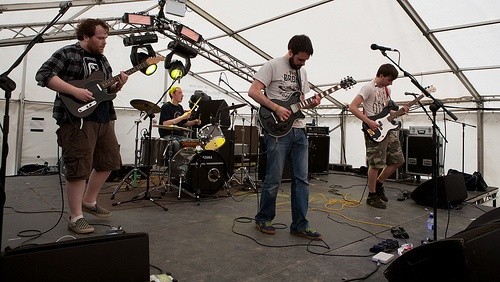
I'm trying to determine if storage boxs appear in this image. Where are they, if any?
[233,144,249,155]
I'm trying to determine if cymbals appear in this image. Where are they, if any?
[152,125,194,132]
[227,103,247,111]
[129,99,161,113]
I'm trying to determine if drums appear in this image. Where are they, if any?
[140,137,171,167]
[171,147,227,196]
[197,124,226,151]
[182,139,202,149]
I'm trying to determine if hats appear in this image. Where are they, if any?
[169,86,181,95]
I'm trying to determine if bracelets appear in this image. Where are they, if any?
[274,106,280,111]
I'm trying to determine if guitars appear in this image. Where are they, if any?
[58,55,165,117]
[258,75,358,138]
[362,85,436,144]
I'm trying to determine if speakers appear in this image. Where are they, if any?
[383,207,500,281]
[191,99,231,130]
[0,233,149,282]
[258,135,331,180]
[411,174,468,209]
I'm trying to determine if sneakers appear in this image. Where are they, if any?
[377,186,388,202]
[256,221,275,234]
[67,216,94,233]
[81,201,112,218]
[290,226,324,240]
[366,196,386,209]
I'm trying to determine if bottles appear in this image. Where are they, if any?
[427,215,435,242]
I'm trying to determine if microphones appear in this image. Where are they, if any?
[59,1,72,8]
[371,44,398,52]
[178,71,181,83]
[405,92,419,95]
[218,73,222,85]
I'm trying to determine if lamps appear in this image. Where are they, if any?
[164,49,191,80]
[121,12,153,25]
[175,24,203,43]
[129,44,157,75]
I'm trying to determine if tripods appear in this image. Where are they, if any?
[221,109,258,194]
[110,77,196,211]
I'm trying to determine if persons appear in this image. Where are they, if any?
[35,18,128,234]
[350,63,409,208]
[248,35,323,240]
[159,87,201,152]
